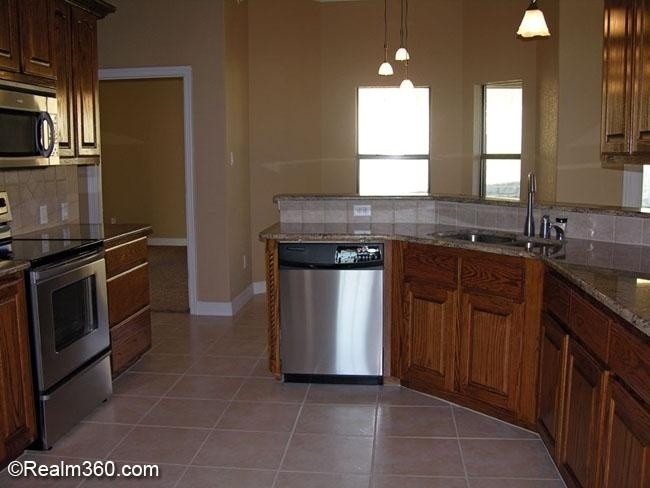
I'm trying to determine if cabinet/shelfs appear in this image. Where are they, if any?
[0,256,38,471]
[0,1,118,169]
[599,0,650,169]
[258,192,650,488]
[12,221,153,380]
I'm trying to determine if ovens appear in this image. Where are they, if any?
[10,240,114,452]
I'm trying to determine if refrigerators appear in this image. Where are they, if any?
[279,240,384,386]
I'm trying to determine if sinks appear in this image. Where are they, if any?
[506,239,563,258]
[426,227,524,243]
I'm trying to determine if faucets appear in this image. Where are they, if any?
[524,171,539,241]
[548,224,564,239]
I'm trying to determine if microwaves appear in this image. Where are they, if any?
[0,79,60,168]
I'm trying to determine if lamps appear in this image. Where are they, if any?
[377,0,415,92]
[515,0,551,38]
